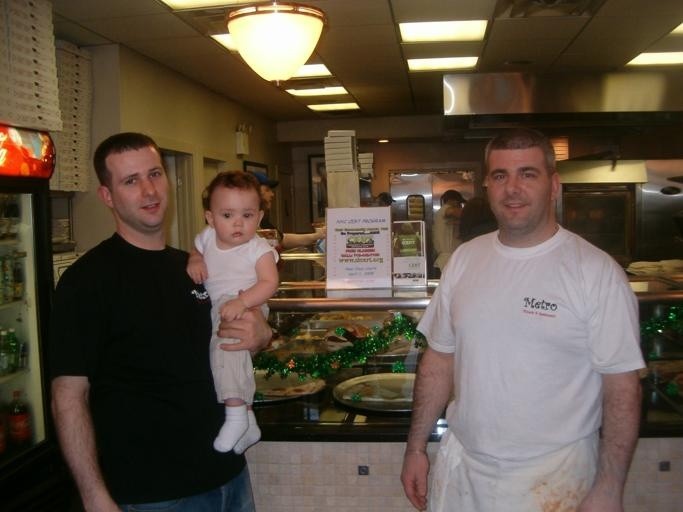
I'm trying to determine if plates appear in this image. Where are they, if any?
[249,366,327,404]
[331,372,418,413]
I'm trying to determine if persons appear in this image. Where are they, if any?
[182,168,283,457]
[449,196,499,258]
[43,131,281,512]
[427,189,466,278]
[245,169,327,251]
[374,190,403,222]
[396,125,649,511]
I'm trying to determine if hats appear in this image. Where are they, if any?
[443,190,468,203]
[250,170,279,188]
[379,192,397,203]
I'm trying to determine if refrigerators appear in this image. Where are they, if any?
[0,122,72,512]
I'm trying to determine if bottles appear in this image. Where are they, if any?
[1,192,36,466]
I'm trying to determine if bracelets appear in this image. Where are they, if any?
[267,321,279,355]
[404,448,427,455]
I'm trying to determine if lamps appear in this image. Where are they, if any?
[222,1,330,88]
